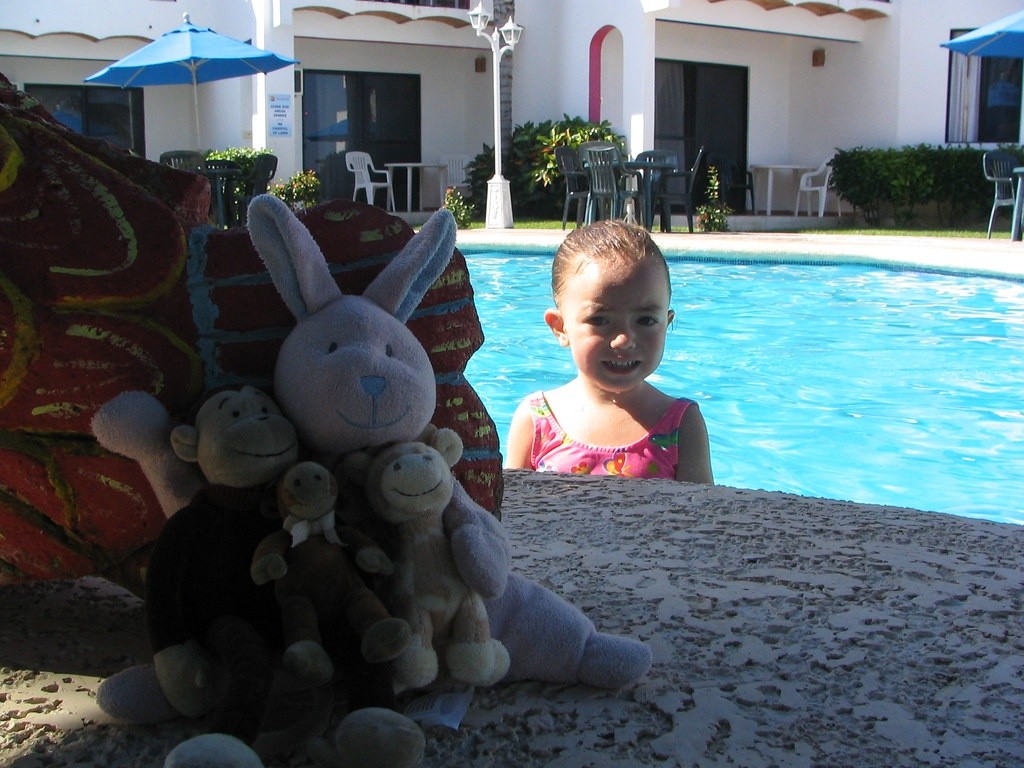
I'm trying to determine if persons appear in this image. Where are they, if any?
[501,219,717,484]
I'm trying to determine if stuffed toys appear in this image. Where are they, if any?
[94,185,655,767]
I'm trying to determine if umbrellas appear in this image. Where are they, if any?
[83,13,302,155]
[939,6,1024,58]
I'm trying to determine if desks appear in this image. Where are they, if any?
[196,168,242,227]
[751,164,818,216]
[585,160,678,233]
[1011,167,1024,240]
[383,162,447,213]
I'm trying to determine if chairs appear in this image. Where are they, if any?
[345,151,396,213]
[619,150,667,235]
[706,153,754,216]
[160,149,278,227]
[440,155,473,205]
[554,146,606,230]
[578,141,647,229]
[982,149,1020,239]
[794,153,842,217]
[652,146,705,232]
